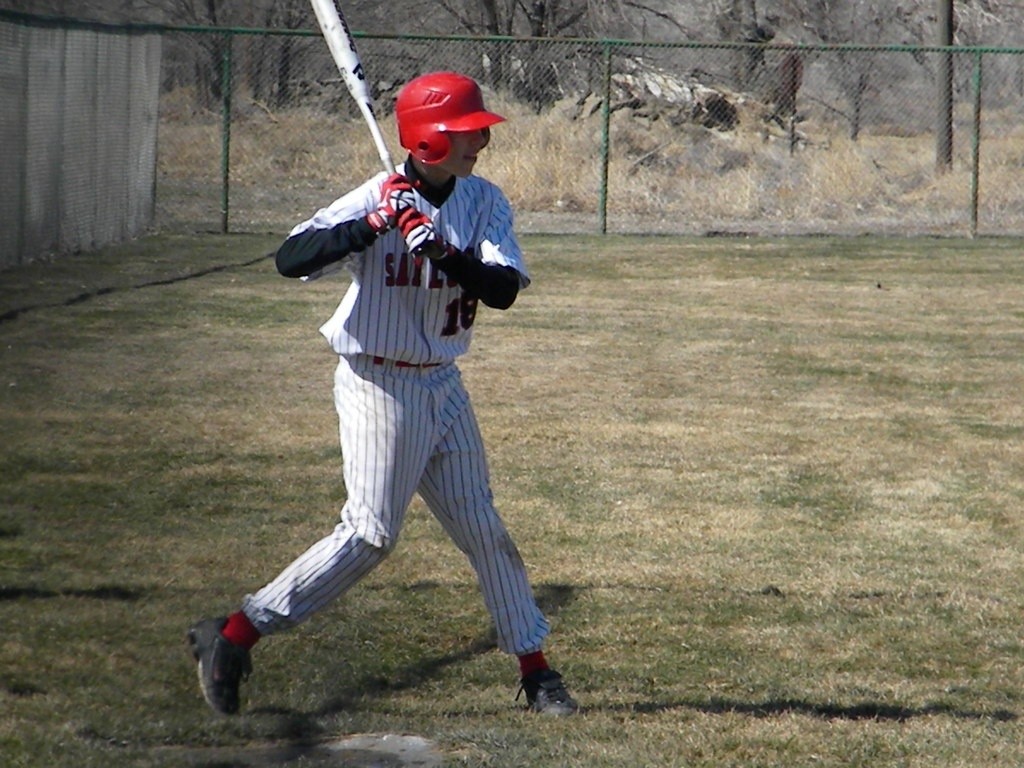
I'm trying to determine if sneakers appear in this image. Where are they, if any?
[515,669,578,716]
[185,616,251,715]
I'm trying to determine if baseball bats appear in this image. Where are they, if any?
[310,0,428,259]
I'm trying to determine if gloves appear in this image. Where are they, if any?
[398,207,443,266]
[367,173,421,235]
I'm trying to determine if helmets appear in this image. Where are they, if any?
[396,71,507,165]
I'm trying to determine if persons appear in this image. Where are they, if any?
[189,71,581,719]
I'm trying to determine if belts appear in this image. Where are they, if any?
[374,356,441,367]
